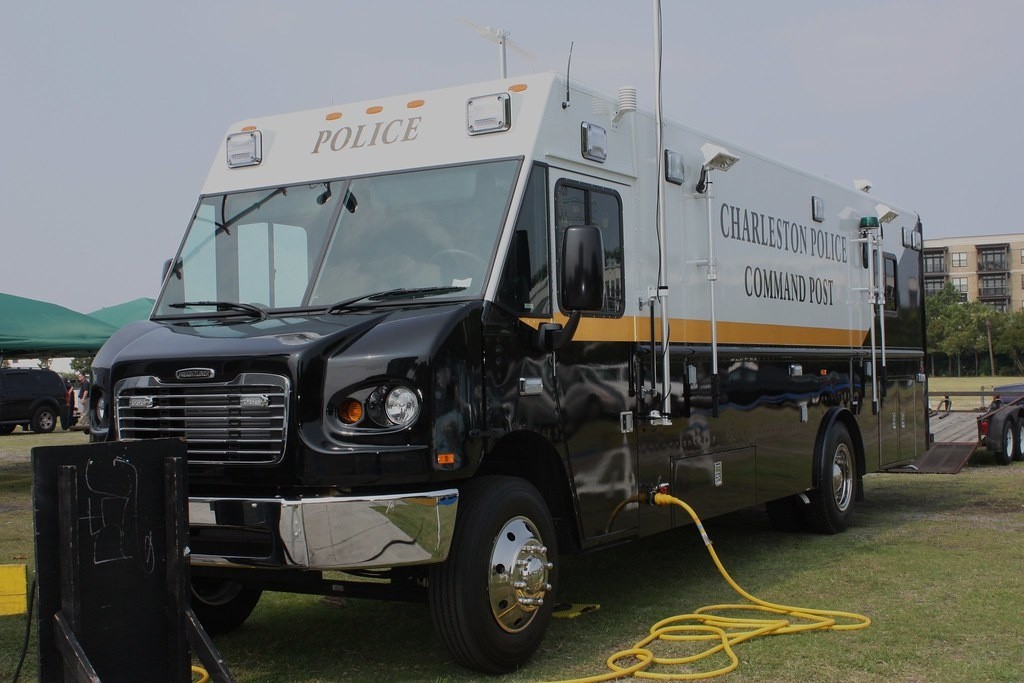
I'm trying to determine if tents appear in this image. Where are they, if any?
[0,292,198,360]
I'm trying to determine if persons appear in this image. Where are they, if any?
[63,372,90,432]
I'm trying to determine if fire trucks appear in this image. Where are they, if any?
[83,0,935,682]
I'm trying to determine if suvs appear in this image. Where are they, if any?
[0,366,75,435]
